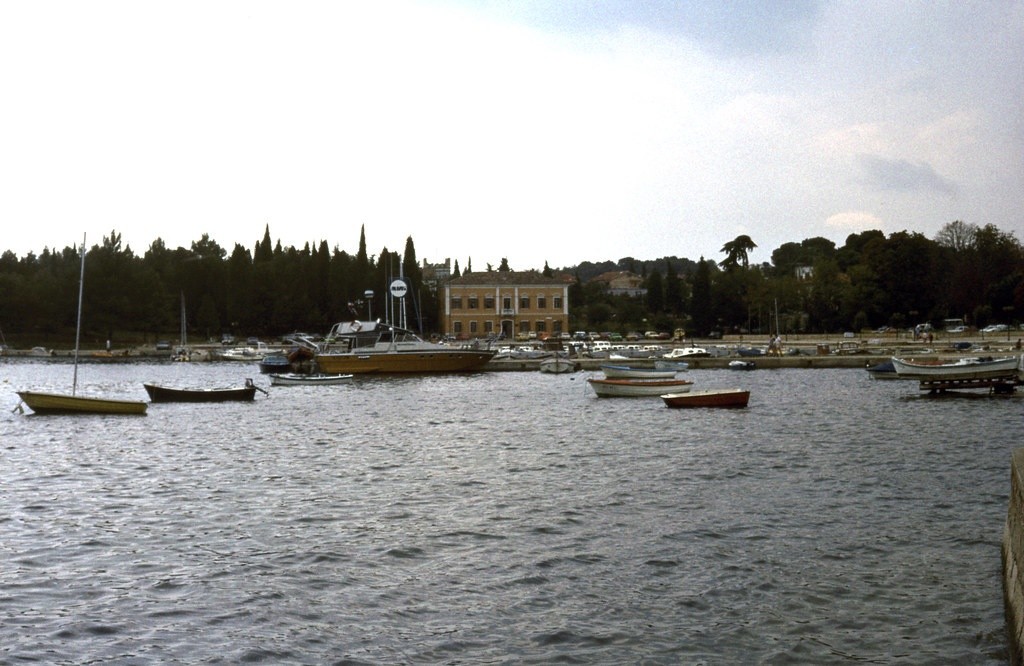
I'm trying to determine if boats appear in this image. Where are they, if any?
[223,342,287,361]
[309,328,497,373]
[865,360,902,380]
[886,353,1018,380]
[266,369,355,388]
[539,358,580,378]
[584,377,695,400]
[599,365,677,379]
[492,340,799,358]
[258,355,289,373]
[660,385,752,408]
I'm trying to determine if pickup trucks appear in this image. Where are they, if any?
[282,332,315,342]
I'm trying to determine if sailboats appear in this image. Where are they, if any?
[15,225,150,417]
[139,290,264,404]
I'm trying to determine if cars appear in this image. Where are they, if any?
[514,331,585,340]
[979,322,1009,334]
[659,332,670,340]
[625,334,638,342]
[606,332,622,340]
[221,335,240,345]
[645,332,660,339]
[246,337,259,345]
[157,340,172,350]
[586,330,601,341]
[947,326,968,333]
